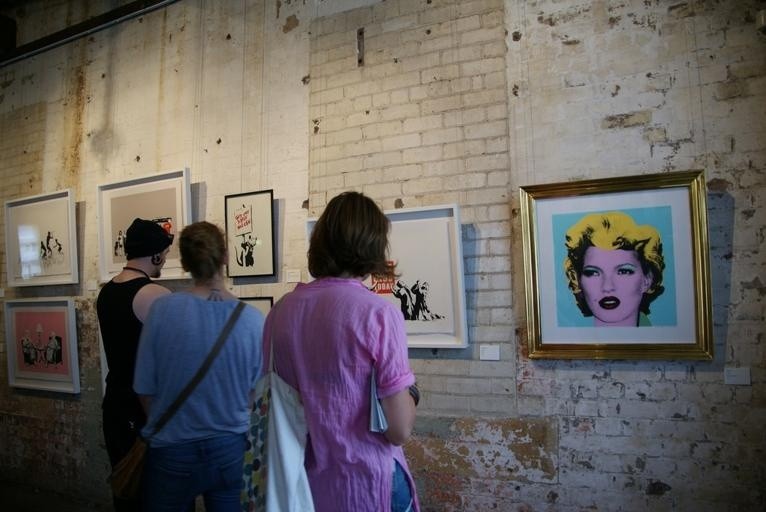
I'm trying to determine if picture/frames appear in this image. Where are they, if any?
[95,167,193,285]
[5,296,80,394]
[518,167,715,362]
[305,202,469,350]
[224,188,274,317]
[5,187,78,287]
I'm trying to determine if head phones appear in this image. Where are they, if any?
[152,253,162,265]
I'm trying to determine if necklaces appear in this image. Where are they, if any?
[123,267,150,279]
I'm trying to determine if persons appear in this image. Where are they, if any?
[263,192,420,512]
[563,210,664,327]
[40,231,62,259]
[132,222,267,512]
[97,218,173,512]
[390,281,446,322]
[21,328,63,370]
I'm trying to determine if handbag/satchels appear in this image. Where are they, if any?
[106,436,151,503]
[238,293,317,511]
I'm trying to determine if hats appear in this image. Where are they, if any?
[125,217,175,261]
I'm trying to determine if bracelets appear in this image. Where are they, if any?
[409,386,420,405]
